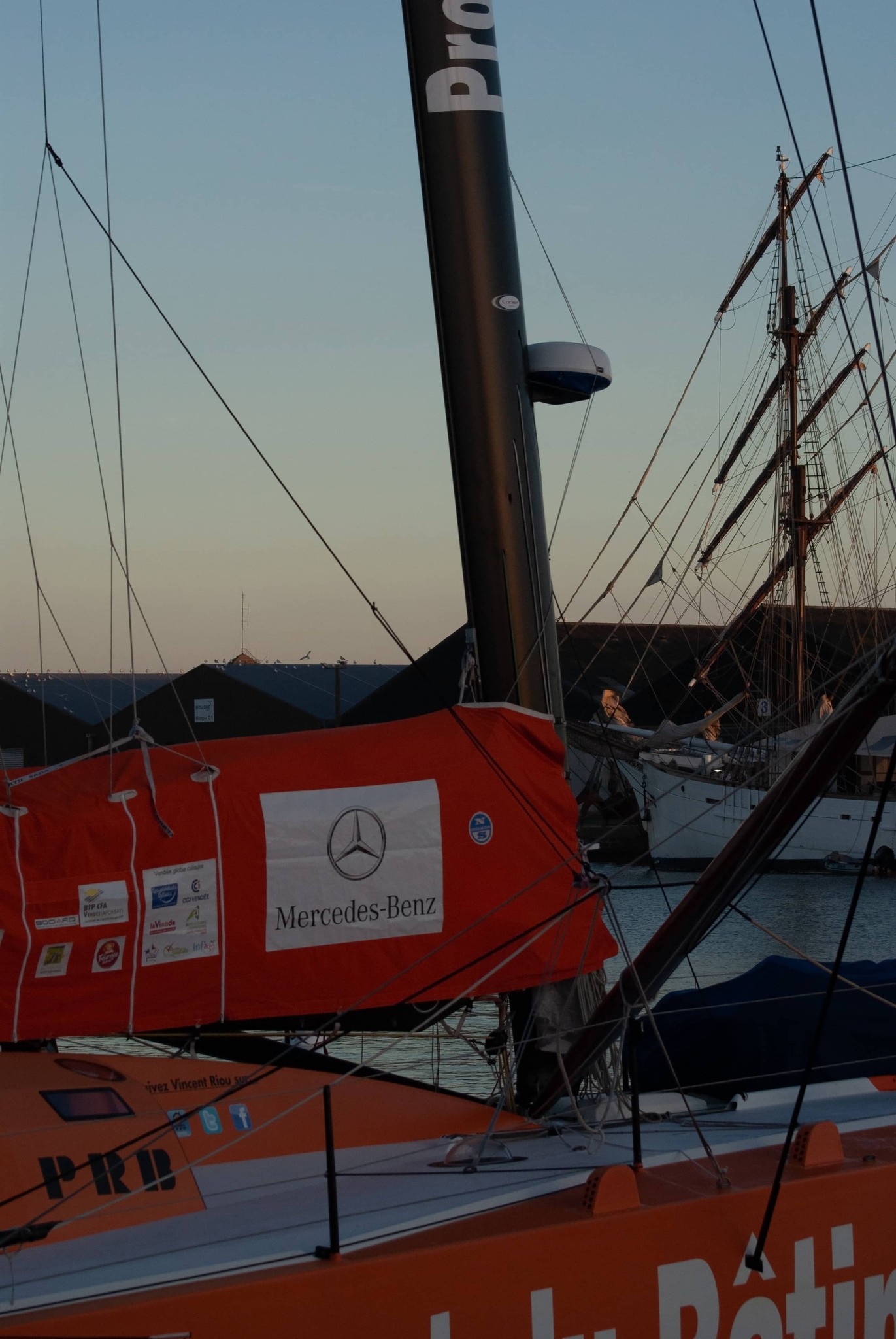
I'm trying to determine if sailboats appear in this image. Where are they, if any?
[2,0,894,1339]
